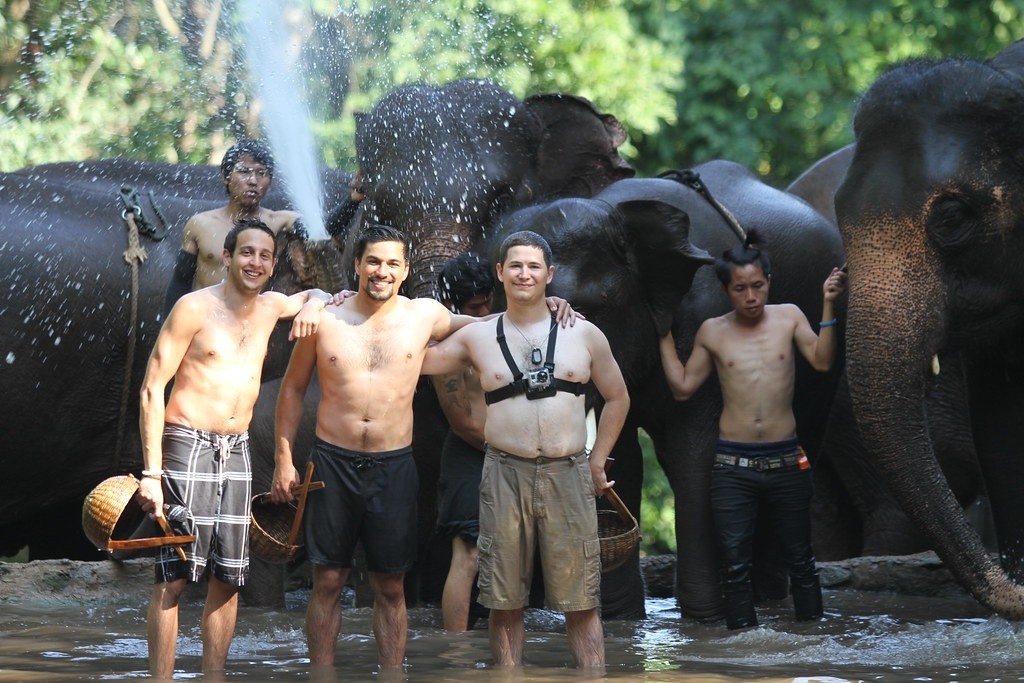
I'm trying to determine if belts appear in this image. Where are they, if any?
[715,451,802,472]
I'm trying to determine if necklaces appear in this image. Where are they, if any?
[506,310,557,364]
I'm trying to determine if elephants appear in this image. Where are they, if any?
[0,37,1024,625]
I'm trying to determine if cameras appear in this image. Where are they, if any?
[527,368,551,388]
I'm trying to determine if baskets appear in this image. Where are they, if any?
[81,472,196,561]
[247,462,326,565]
[597,487,643,573]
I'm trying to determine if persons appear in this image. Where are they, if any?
[270,226,586,667]
[325,230,631,668]
[139,219,331,681]
[659,227,848,630]
[164,140,372,359]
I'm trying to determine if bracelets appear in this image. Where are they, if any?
[142,470,164,475]
[819,318,836,327]
